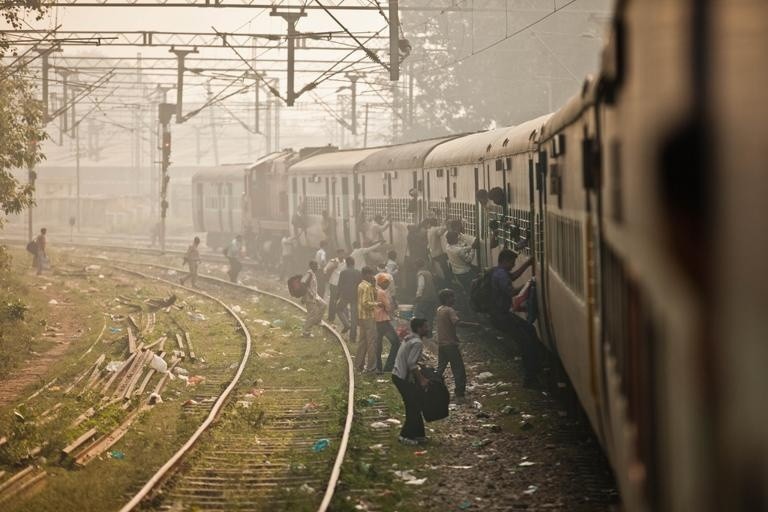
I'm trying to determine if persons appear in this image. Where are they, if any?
[489,248,561,391]
[34,228,47,275]
[392,317,432,446]
[279,208,480,372]
[434,288,482,404]
[226,234,244,283]
[180,236,202,290]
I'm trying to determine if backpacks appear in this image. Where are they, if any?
[287,273,313,299]
[469,266,504,313]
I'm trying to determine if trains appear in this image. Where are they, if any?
[184,3,767,509]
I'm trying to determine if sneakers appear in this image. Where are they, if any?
[341,324,350,333]
[354,367,393,376]
[398,435,430,445]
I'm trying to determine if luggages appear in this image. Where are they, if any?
[415,364,450,422]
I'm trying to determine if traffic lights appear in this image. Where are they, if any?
[162,131,171,156]
